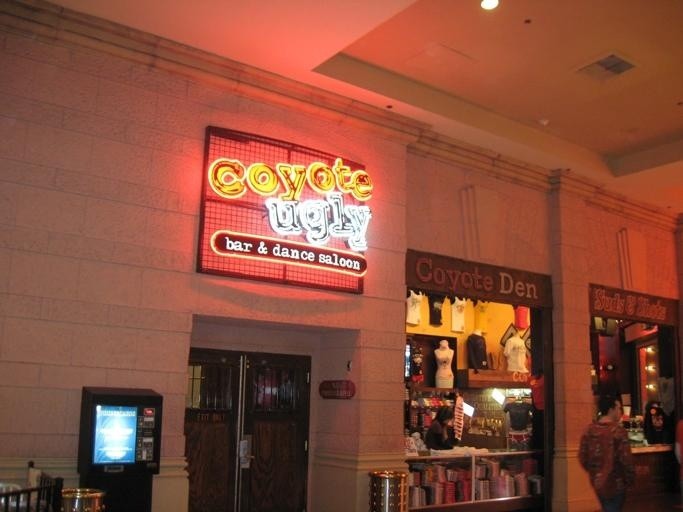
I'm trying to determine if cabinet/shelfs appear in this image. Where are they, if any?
[405,448,545,512]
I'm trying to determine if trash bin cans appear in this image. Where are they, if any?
[368,470,409,512]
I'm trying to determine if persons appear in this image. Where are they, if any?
[673,419,682,491]
[424,405,454,450]
[577,393,635,512]
[528,366,544,413]
[502,394,536,439]
[467,328,488,370]
[502,331,527,371]
[432,339,453,388]
